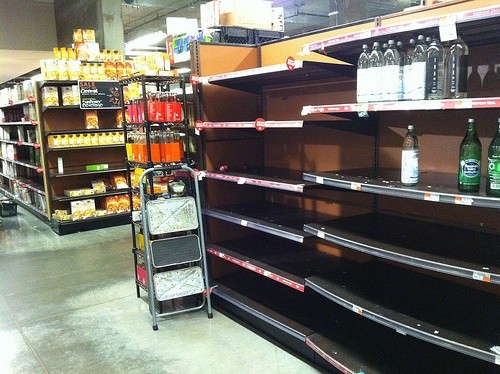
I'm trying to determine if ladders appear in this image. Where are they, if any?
[138,165,213,330]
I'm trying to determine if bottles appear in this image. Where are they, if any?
[445,32,469,98]
[369,35,432,103]
[458,118,483,193]
[126,129,184,163]
[356,44,371,103]
[128,91,182,123]
[427,34,445,100]
[486,118,500,198]
[401,125,420,186]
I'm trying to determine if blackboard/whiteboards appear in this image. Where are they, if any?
[77,80,122,109]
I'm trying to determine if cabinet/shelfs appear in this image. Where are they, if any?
[190,0,500,374]
[36,79,136,235]
[118,73,200,317]
[0,67,51,227]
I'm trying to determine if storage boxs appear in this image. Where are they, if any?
[42,84,80,108]
[48,131,125,148]
[84,110,99,129]
[52,174,141,222]
[116,109,130,128]
[85,164,108,171]
[0,198,18,218]
